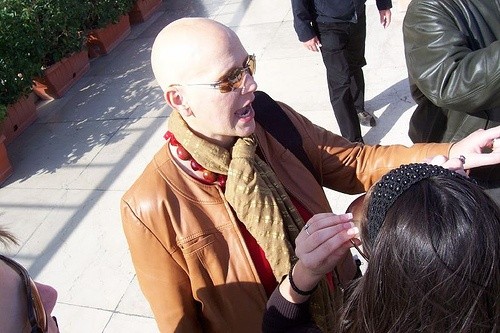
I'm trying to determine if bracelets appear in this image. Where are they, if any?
[289,263,320,296]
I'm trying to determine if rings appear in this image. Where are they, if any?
[304,224,311,235]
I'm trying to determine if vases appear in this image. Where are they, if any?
[130,0,162,22]
[0,133,12,187]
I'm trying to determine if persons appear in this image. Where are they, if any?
[120,17,500,333]
[263,155,500,333]
[402,0,500,155]
[291,0,393,144]
[0,226,60,333]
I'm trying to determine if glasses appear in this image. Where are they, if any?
[0,254,48,333]
[169,53,256,93]
[345,194,369,262]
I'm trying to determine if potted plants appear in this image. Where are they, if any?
[0,33,38,147]
[74,0,132,57]
[0,0,91,101]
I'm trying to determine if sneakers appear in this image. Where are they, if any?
[357,111,376,127]
